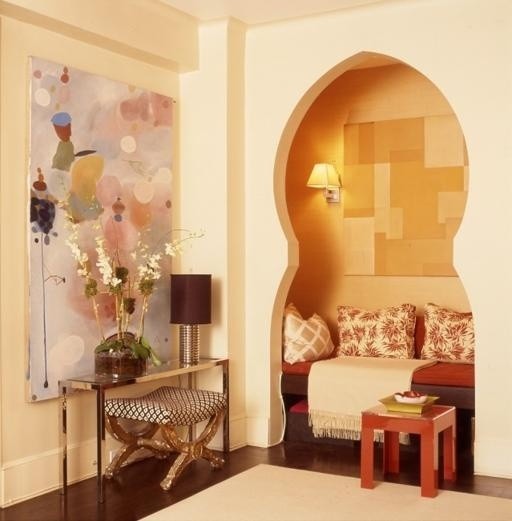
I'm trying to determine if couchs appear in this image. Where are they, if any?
[281,313,474,408]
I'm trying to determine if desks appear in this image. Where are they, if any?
[50,356,237,504]
[356,405,460,498]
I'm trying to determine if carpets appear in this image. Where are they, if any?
[139,462,511,520]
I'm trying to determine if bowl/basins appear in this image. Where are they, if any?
[394,392,428,404]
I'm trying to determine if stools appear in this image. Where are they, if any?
[105,388,228,494]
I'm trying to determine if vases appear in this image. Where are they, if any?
[90,345,151,377]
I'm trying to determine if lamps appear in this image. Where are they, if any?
[306,159,340,204]
[171,273,210,366]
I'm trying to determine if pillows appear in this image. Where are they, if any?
[281,299,475,369]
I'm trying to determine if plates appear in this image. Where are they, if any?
[380,391,439,414]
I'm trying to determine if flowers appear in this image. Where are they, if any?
[49,187,210,354]
